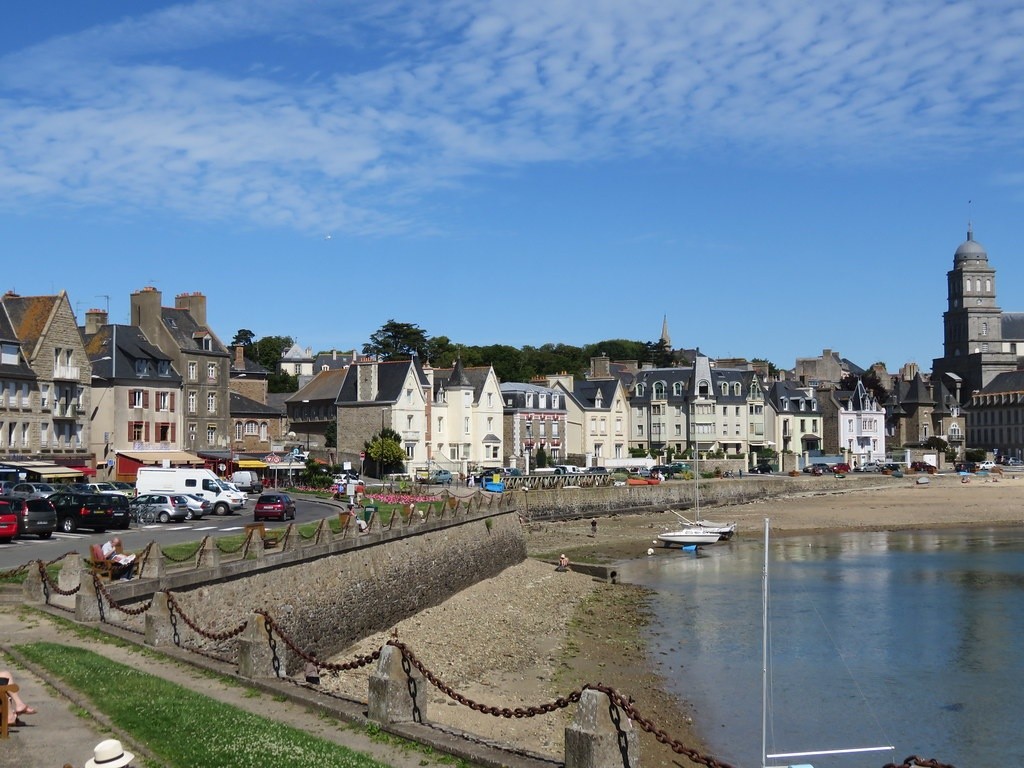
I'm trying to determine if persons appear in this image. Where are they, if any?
[355,481,367,509]
[558,554,568,570]
[854,458,858,468]
[591,518,597,537]
[0,670,35,724]
[466,474,475,489]
[738,468,743,479]
[85,738,134,768]
[101,537,134,582]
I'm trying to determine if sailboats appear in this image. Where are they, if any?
[657,440,721,543]
[671,442,738,541]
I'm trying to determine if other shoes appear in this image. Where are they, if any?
[130,578,134,581]
[120,577,129,581]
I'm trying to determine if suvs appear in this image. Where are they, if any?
[233,471,262,493]
[554,464,582,475]
[668,462,691,473]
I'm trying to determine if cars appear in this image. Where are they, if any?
[253,493,297,521]
[609,468,630,476]
[749,463,774,475]
[332,474,364,485]
[629,465,651,477]
[420,469,453,484]
[466,466,520,483]
[226,481,249,507]
[803,454,1023,473]
[0,476,137,542]
[127,492,188,522]
[181,494,211,519]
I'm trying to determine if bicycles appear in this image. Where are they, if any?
[131,503,155,526]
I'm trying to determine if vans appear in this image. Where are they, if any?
[134,467,242,516]
[649,465,673,475]
[584,466,607,477]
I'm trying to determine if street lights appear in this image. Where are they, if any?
[380,407,389,479]
